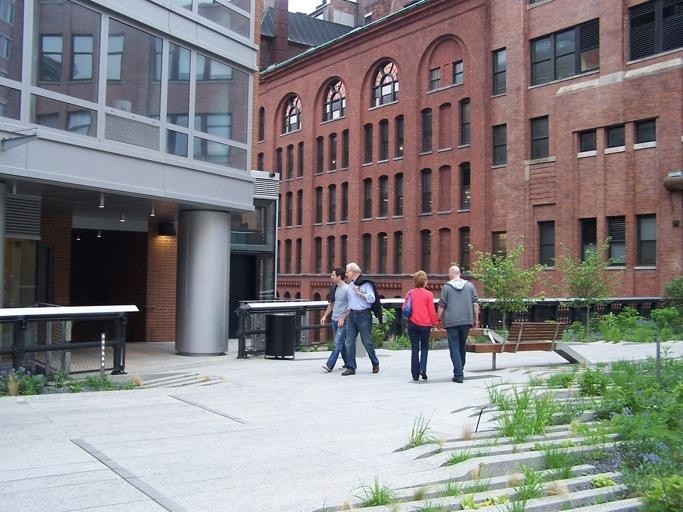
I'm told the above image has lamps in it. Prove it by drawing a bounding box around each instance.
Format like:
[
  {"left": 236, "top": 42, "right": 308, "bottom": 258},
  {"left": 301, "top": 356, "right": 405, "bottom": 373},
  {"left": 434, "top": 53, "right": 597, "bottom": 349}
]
[{"left": 76, "top": 191, "right": 157, "bottom": 241}]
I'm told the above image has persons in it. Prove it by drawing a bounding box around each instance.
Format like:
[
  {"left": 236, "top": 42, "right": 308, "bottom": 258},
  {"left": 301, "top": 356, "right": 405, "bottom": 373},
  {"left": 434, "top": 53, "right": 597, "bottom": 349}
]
[
  {"left": 339, "top": 262, "right": 382, "bottom": 376},
  {"left": 437, "top": 266, "right": 479, "bottom": 383},
  {"left": 400, "top": 270, "right": 437, "bottom": 382},
  {"left": 319, "top": 266, "right": 347, "bottom": 373}
]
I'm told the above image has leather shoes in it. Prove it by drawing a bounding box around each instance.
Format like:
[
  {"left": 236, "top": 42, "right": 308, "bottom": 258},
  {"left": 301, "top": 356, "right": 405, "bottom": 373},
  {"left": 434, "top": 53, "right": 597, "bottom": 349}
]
[{"left": 373, "top": 363, "right": 379, "bottom": 372}]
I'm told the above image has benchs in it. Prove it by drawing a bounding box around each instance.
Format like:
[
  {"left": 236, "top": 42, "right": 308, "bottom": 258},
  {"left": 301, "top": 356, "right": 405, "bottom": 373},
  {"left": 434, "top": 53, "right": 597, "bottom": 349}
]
[
  {"left": 463, "top": 322, "right": 566, "bottom": 373},
  {"left": 427, "top": 327, "right": 484, "bottom": 350}
]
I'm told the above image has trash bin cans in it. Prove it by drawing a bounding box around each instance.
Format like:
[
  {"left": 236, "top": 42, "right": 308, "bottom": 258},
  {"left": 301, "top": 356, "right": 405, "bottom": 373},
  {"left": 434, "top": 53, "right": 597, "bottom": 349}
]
[{"left": 264, "top": 313, "right": 296, "bottom": 359}]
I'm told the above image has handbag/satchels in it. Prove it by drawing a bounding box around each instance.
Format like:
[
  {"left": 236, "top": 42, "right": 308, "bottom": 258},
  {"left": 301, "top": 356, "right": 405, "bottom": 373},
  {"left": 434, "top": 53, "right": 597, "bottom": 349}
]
[{"left": 402, "top": 297, "right": 412, "bottom": 317}]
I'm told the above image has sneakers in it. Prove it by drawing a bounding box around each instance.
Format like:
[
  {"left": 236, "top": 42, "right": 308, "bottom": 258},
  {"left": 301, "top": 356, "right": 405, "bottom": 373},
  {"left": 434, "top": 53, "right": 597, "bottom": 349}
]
[
  {"left": 419, "top": 368, "right": 426, "bottom": 379},
  {"left": 321, "top": 363, "right": 355, "bottom": 375}
]
